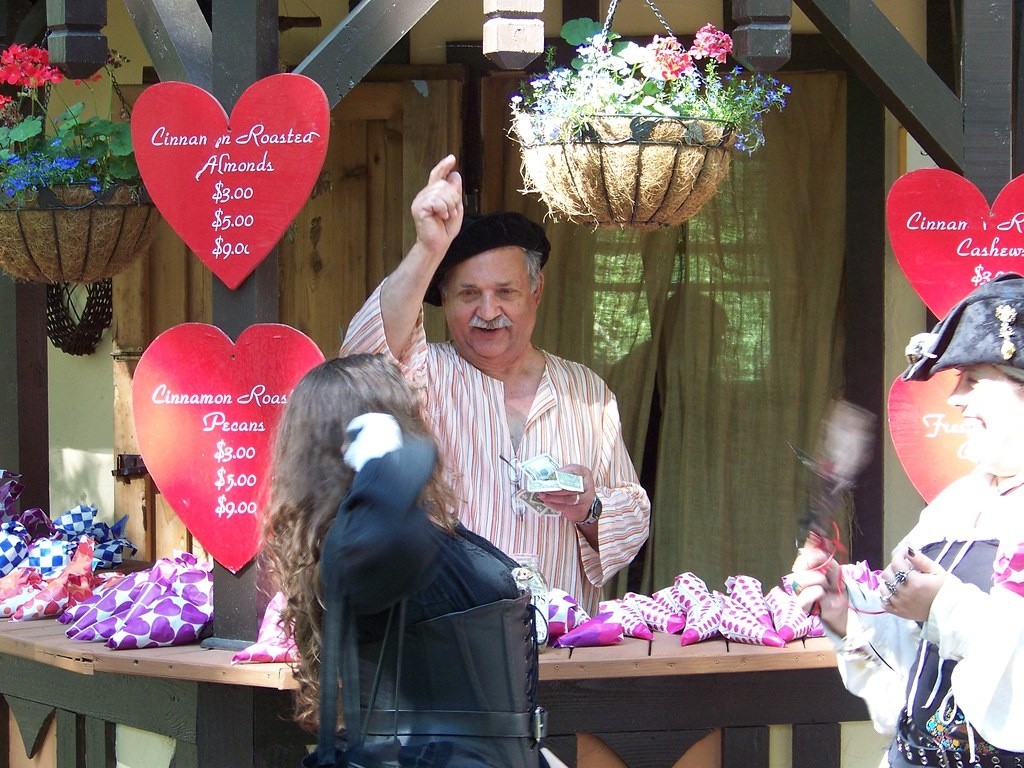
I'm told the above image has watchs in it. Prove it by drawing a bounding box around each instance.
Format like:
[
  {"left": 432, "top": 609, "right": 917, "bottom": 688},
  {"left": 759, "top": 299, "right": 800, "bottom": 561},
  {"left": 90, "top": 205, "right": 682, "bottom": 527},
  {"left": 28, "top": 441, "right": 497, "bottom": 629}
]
[{"left": 575, "top": 495, "right": 602, "bottom": 525}]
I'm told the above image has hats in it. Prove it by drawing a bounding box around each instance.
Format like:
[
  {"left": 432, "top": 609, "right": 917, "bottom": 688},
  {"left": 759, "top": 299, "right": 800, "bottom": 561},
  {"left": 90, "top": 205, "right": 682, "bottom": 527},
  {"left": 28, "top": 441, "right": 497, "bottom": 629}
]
[
  {"left": 421, "top": 211, "right": 551, "bottom": 307},
  {"left": 902, "top": 273, "right": 1024, "bottom": 382}
]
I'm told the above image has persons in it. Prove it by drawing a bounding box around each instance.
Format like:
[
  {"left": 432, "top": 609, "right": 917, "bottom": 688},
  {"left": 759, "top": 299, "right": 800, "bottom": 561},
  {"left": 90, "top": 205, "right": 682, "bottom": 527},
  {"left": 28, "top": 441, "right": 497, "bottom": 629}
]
[
  {"left": 340, "top": 155, "right": 652, "bottom": 767},
  {"left": 268, "top": 353, "right": 545, "bottom": 768},
  {"left": 792, "top": 273, "right": 1024, "bottom": 768}
]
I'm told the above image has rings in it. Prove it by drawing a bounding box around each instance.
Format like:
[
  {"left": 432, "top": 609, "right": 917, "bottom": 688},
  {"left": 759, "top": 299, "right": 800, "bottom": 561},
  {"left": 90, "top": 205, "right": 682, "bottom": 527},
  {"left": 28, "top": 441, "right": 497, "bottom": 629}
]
[
  {"left": 895, "top": 570, "right": 911, "bottom": 583},
  {"left": 885, "top": 581, "right": 897, "bottom": 593},
  {"left": 880, "top": 593, "right": 893, "bottom": 605},
  {"left": 572, "top": 495, "right": 579, "bottom": 506},
  {"left": 792, "top": 581, "right": 803, "bottom": 594}
]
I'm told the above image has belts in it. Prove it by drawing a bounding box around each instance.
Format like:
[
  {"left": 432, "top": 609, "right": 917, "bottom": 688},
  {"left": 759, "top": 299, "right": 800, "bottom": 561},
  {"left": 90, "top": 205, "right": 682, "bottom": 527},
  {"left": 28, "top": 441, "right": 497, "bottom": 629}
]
[{"left": 359, "top": 707, "right": 549, "bottom": 750}]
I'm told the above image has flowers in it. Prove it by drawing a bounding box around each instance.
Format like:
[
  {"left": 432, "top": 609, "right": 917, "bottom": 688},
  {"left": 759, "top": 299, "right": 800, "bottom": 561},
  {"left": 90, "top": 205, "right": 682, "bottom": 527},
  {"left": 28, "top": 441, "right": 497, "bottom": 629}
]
[
  {"left": 0, "top": 40, "right": 162, "bottom": 282},
  {"left": 505, "top": 16, "right": 793, "bottom": 229}
]
